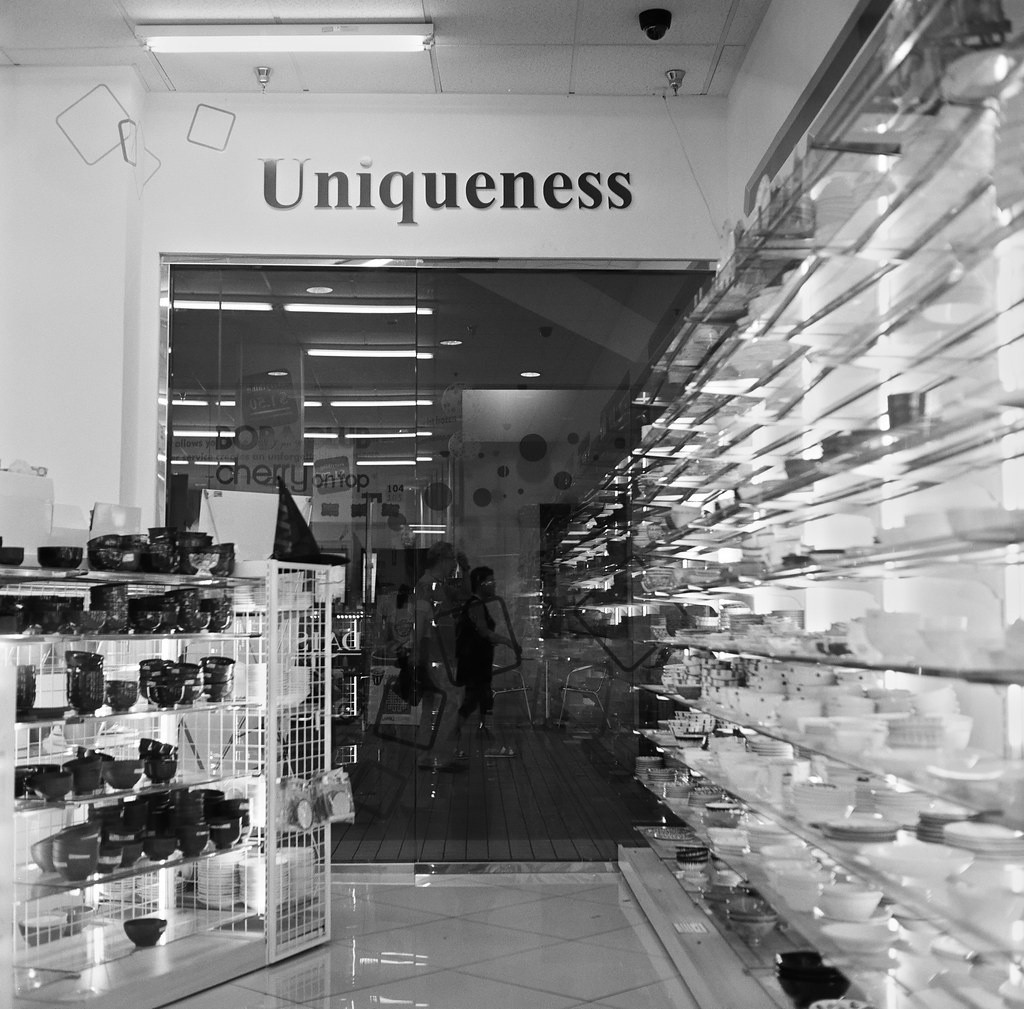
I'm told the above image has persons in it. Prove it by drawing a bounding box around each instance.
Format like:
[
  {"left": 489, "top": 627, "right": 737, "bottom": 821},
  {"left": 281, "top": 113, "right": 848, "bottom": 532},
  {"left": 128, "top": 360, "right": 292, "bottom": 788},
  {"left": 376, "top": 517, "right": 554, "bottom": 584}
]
[
  {"left": 453, "top": 567, "right": 523, "bottom": 761},
  {"left": 383, "top": 584, "right": 413, "bottom": 656},
  {"left": 408, "top": 541, "right": 473, "bottom": 772}
]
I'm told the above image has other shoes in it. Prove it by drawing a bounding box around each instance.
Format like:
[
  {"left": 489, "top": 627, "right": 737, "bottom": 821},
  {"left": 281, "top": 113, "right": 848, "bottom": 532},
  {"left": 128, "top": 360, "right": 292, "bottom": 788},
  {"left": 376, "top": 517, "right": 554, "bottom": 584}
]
[
  {"left": 434, "top": 755, "right": 470, "bottom": 777},
  {"left": 417, "top": 752, "right": 441, "bottom": 771}
]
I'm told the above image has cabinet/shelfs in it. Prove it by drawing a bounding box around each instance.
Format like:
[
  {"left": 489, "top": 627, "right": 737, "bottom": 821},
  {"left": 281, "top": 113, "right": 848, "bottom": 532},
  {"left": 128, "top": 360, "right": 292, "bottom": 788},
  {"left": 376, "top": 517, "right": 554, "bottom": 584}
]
[
  {"left": 538, "top": 440, "right": 640, "bottom": 633},
  {"left": 608, "top": 0, "right": 1023, "bottom": 1009},
  {"left": 1, "top": 556, "right": 335, "bottom": 1009}
]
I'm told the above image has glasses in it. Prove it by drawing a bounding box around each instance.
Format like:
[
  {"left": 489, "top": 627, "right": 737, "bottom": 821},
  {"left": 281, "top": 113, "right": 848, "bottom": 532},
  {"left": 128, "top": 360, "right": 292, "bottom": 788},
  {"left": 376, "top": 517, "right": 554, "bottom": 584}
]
[
  {"left": 438, "top": 553, "right": 458, "bottom": 561},
  {"left": 479, "top": 578, "right": 499, "bottom": 588}
]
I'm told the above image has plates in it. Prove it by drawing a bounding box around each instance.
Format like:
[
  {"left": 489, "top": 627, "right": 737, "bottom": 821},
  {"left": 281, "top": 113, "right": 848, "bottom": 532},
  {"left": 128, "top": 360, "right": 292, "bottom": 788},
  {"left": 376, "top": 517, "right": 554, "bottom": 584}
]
[{"left": 196, "top": 560, "right": 319, "bottom": 913}]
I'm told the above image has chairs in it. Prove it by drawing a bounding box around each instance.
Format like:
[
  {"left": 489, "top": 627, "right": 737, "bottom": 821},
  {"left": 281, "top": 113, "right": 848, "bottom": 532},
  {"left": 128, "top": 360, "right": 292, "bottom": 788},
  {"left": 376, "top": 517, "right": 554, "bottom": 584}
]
[
  {"left": 492, "top": 664, "right": 535, "bottom": 727},
  {"left": 558, "top": 658, "right": 613, "bottom": 730}
]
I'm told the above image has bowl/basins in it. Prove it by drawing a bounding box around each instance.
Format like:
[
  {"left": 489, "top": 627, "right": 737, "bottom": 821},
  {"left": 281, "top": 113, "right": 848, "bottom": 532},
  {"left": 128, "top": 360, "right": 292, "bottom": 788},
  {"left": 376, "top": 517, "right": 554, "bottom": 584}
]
[
  {"left": 650, "top": 2, "right": 1023, "bottom": 1009},
  {"left": 0, "top": 526, "right": 252, "bottom": 949}
]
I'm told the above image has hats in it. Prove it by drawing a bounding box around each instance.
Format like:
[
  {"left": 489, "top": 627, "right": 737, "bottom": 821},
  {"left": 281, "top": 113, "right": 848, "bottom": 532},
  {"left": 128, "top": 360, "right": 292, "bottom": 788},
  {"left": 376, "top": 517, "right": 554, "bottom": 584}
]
[{"left": 420, "top": 540, "right": 455, "bottom": 567}]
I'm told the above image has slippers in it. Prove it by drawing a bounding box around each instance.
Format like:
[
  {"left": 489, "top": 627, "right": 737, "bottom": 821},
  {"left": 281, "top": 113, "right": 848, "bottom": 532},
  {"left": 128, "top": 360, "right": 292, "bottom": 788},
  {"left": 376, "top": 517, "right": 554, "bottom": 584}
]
[
  {"left": 483, "top": 743, "right": 522, "bottom": 760},
  {"left": 454, "top": 745, "right": 482, "bottom": 760}
]
